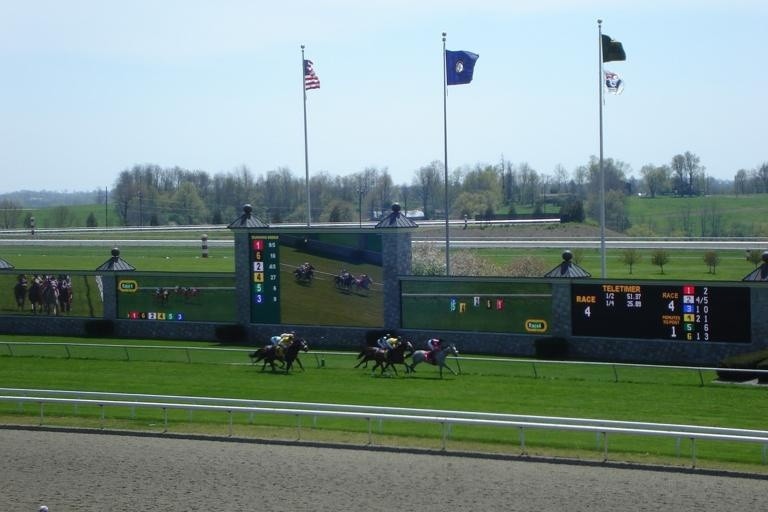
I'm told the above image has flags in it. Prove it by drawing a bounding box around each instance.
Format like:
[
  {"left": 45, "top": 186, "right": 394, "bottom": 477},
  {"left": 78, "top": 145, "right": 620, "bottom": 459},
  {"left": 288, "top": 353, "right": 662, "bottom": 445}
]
[
  {"left": 446, "top": 50, "right": 479, "bottom": 85},
  {"left": 602, "top": 34, "right": 626, "bottom": 63},
  {"left": 304, "top": 59, "right": 320, "bottom": 90},
  {"left": 604, "top": 72, "right": 625, "bottom": 96}
]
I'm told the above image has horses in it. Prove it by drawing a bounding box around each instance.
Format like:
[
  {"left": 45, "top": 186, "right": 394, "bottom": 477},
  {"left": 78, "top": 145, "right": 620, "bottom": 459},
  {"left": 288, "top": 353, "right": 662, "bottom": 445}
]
[
  {"left": 407, "top": 343, "right": 458, "bottom": 379},
  {"left": 249, "top": 337, "right": 309, "bottom": 375},
  {"left": 334, "top": 274, "right": 374, "bottom": 296},
  {"left": 354, "top": 339, "right": 415, "bottom": 375},
  {"left": 13, "top": 278, "right": 73, "bottom": 317},
  {"left": 292, "top": 264, "right": 316, "bottom": 285}
]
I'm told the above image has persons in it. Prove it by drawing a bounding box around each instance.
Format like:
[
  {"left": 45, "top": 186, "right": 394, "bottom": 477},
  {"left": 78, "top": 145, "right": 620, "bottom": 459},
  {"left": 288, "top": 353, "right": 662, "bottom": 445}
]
[
  {"left": 377, "top": 333, "right": 402, "bottom": 361},
  {"left": 334, "top": 268, "right": 370, "bottom": 290},
  {"left": 296, "top": 260, "right": 311, "bottom": 280},
  {"left": 12, "top": 274, "right": 73, "bottom": 301},
  {"left": 263, "top": 329, "right": 298, "bottom": 363},
  {"left": 427, "top": 338, "right": 444, "bottom": 365}
]
